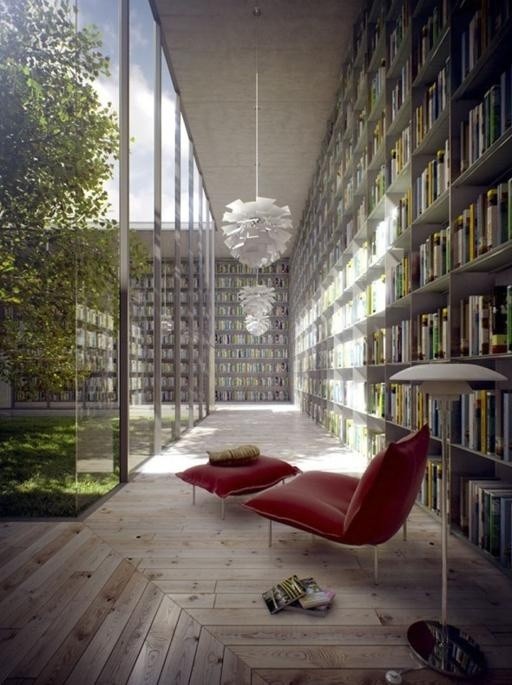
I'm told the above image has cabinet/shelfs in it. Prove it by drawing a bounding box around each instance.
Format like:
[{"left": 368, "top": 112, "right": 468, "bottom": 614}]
[
  {"left": 41, "top": 255, "right": 206, "bottom": 408},
  {"left": 214, "top": 257, "right": 289, "bottom": 402},
  {"left": 288, "top": 1, "right": 512, "bottom": 575}
]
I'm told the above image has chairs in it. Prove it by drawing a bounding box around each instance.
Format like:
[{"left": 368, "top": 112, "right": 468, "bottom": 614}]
[{"left": 241, "top": 423, "right": 432, "bottom": 585}]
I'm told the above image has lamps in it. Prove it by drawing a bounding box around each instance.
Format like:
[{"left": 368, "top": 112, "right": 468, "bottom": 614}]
[
  {"left": 239, "top": 106, "right": 277, "bottom": 318},
  {"left": 388, "top": 364, "right": 509, "bottom": 678},
  {"left": 222, "top": 7, "right": 293, "bottom": 268},
  {"left": 244, "top": 266, "right": 272, "bottom": 335}
]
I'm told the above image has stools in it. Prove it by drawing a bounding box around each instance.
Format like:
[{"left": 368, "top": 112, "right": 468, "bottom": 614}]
[{"left": 175, "top": 445, "right": 302, "bottom": 520}]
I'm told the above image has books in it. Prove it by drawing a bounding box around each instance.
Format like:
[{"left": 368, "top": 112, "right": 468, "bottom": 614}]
[
  {"left": 369, "top": 164, "right": 389, "bottom": 211},
  {"left": 391, "top": 57, "right": 412, "bottom": 123},
  {"left": 311, "top": 106, "right": 352, "bottom": 184},
  {"left": 261, "top": 573, "right": 334, "bottom": 616},
  {"left": 352, "top": 422, "right": 368, "bottom": 455},
  {"left": 367, "top": 428, "right": 385, "bottom": 459},
  {"left": 354, "top": 106, "right": 368, "bottom": 142},
  {"left": 413, "top": 383, "right": 451, "bottom": 440},
  {"left": 416, "top": 305, "right": 447, "bottom": 361},
  {"left": 420, "top": 454, "right": 442, "bottom": 520},
  {"left": 215, "top": 389, "right": 288, "bottom": 401},
  {"left": 287, "top": 241, "right": 352, "bottom": 433},
  {"left": 369, "top": 328, "right": 385, "bottom": 365},
  {"left": 354, "top": 289, "right": 370, "bottom": 322},
  {"left": 388, "top": 190, "right": 412, "bottom": 244},
  {"left": 215, "top": 318, "right": 286, "bottom": 330},
  {"left": 216, "top": 275, "right": 287, "bottom": 287},
  {"left": 369, "top": 57, "right": 388, "bottom": 110},
  {"left": 460, "top": 389, "right": 510, "bottom": 462},
  {"left": 370, "top": 273, "right": 386, "bottom": 313},
  {"left": 388, "top": 382, "right": 413, "bottom": 429},
  {"left": 215, "top": 305, "right": 286, "bottom": 316},
  {"left": 6, "top": 259, "right": 207, "bottom": 407},
  {"left": 367, "top": 382, "right": 385, "bottom": 418},
  {"left": 390, "top": 120, "right": 413, "bottom": 184},
  {"left": 414, "top": 58, "right": 450, "bottom": 148},
  {"left": 452, "top": 1, "right": 511, "bottom": 86},
  {"left": 414, "top": 140, "right": 448, "bottom": 217},
  {"left": 352, "top": 333, "right": 366, "bottom": 367},
  {"left": 416, "top": 1, "right": 449, "bottom": 64},
  {"left": 390, "top": 320, "right": 410, "bottom": 364},
  {"left": 388, "top": 1, "right": 412, "bottom": 66},
  {"left": 337, "top": 15, "right": 367, "bottom": 104},
  {"left": 296, "top": 184, "right": 355, "bottom": 240},
  {"left": 216, "top": 361, "right": 286, "bottom": 373},
  {"left": 215, "top": 332, "right": 287, "bottom": 345},
  {"left": 366, "top": 15, "right": 384, "bottom": 56},
  {"left": 371, "top": 219, "right": 388, "bottom": 265},
  {"left": 368, "top": 114, "right": 387, "bottom": 158},
  {"left": 354, "top": 146, "right": 371, "bottom": 189},
  {"left": 457, "top": 476, "right": 511, "bottom": 568},
  {"left": 389, "top": 256, "right": 409, "bottom": 303},
  {"left": 419, "top": 226, "right": 452, "bottom": 287},
  {"left": 459, "top": 72, "right": 510, "bottom": 174},
  {"left": 351, "top": 382, "right": 366, "bottom": 412},
  {"left": 215, "top": 375, "right": 287, "bottom": 387},
  {"left": 213, "top": 287, "right": 286, "bottom": 301},
  {"left": 356, "top": 193, "right": 370, "bottom": 231},
  {"left": 216, "top": 263, "right": 286, "bottom": 272},
  {"left": 356, "top": 241, "right": 367, "bottom": 277},
  {"left": 216, "top": 347, "right": 288, "bottom": 358},
  {"left": 458, "top": 284, "right": 511, "bottom": 357},
  {"left": 456, "top": 183, "right": 507, "bottom": 266},
  {"left": 343, "top": 418, "right": 353, "bottom": 447}
]
